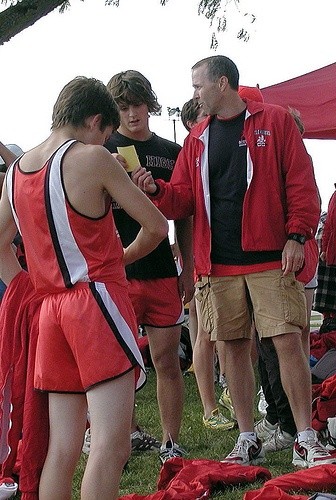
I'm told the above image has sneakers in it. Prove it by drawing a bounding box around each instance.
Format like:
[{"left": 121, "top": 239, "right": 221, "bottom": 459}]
[
  {"left": 82, "top": 427, "right": 162, "bottom": 456},
  {"left": 201, "top": 408, "right": 236, "bottom": 431},
  {"left": 218, "top": 386, "right": 237, "bottom": 420},
  {"left": 221, "top": 435, "right": 268, "bottom": 466},
  {"left": 291, "top": 431, "right": 336, "bottom": 468},
  {"left": 252, "top": 385, "right": 297, "bottom": 454},
  {"left": 159, "top": 442, "right": 185, "bottom": 465}
]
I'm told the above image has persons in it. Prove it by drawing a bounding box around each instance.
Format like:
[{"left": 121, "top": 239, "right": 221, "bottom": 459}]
[
  {"left": 131, "top": 55, "right": 336, "bottom": 469},
  {"left": 0, "top": 74, "right": 170, "bottom": 500},
  {"left": 180, "top": 85, "right": 336, "bottom": 455},
  {"left": 0, "top": 140, "right": 163, "bottom": 500},
  {"left": 106, "top": 70, "right": 195, "bottom": 466}
]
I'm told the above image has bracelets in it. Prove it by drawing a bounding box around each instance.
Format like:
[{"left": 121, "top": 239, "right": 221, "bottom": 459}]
[{"left": 286, "top": 233, "right": 306, "bottom": 246}]
